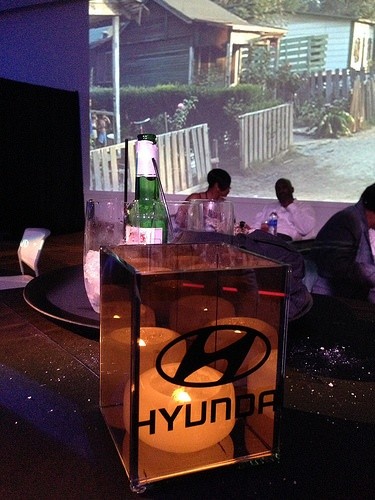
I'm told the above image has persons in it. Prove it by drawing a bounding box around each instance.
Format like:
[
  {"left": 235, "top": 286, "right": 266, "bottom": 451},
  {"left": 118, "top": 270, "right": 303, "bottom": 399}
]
[
  {"left": 252, "top": 177, "right": 314, "bottom": 245},
  {"left": 173, "top": 168, "right": 231, "bottom": 230},
  {"left": 311, "top": 183, "right": 375, "bottom": 303}
]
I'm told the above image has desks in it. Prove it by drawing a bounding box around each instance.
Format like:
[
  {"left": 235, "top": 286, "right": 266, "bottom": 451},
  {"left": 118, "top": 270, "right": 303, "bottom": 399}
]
[{"left": 0, "top": 287, "right": 375, "bottom": 500}]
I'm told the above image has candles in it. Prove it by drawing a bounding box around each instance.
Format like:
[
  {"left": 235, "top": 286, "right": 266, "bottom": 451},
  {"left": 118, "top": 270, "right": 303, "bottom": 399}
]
[{"left": 121, "top": 355, "right": 237, "bottom": 455}]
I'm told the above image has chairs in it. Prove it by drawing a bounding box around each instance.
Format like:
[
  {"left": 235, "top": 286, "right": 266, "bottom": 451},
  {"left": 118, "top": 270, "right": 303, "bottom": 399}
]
[{"left": 0, "top": 228, "right": 51, "bottom": 291}]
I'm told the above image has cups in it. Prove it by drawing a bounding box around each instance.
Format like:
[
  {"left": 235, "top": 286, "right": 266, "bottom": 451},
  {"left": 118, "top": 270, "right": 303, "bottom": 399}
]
[{"left": 83, "top": 193, "right": 235, "bottom": 313}]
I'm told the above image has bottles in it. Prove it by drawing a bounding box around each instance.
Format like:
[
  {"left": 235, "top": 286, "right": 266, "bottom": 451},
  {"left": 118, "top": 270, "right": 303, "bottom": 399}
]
[
  {"left": 123, "top": 133, "right": 168, "bottom": 243},
  {"left": 268, "top": 209, "right": 278, "bottom": 236}
]
